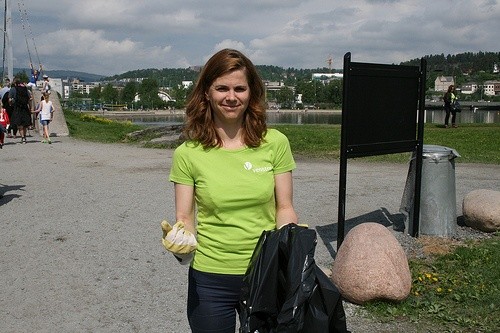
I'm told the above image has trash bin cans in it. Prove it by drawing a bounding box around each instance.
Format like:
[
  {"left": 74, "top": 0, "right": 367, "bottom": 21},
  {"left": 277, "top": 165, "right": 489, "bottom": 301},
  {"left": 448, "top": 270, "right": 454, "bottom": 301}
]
[{"left": 409, "top": 145, "right": 457, "bottom": 241}]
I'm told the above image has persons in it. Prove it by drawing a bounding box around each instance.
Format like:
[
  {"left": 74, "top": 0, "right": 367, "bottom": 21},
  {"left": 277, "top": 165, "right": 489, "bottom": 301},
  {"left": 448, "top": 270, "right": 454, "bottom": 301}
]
[
  {"left": 30, "top": 92, "right": 55, "bottom": 144},
  {"left": 0, "top": 75, "right": 51, "bottom": 149},
  {"left": 29, "top": 63, "right": 42, "bottom": 83},
  {"left": 160, "top": 48, "right": 298, "bottom": 333},
  {"left": 443, "top": 85, "right": 458, "bottom": 128}
]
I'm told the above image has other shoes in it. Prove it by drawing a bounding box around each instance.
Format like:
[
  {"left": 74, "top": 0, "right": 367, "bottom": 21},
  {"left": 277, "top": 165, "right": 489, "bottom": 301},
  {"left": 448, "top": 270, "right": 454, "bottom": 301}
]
[
  {"left": 452, "top": 124, "right": 458, "bottom": 128},
  {"left": 14, "top": 135, "right": 16, "bottom": 139},
  {"left": 47, "top": 139, "right": 52, "bottom": 144},
  {"left": 22, "top": 137, "right": 26, "bottom": 142},
  {"left": 42, "top": 138, "right": 46, "bottom": 143},
  {"left": 6, "top": 133, "right": 12, "bottom": 138},
  {"left": 444, "top": 125, "right": 449, "bottom": 128}
]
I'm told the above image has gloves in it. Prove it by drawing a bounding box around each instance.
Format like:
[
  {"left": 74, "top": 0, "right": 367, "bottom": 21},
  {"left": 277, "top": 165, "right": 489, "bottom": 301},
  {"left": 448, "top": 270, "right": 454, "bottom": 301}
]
[{"left": 161, "top": 220, "right": 198, "bottom": 254}]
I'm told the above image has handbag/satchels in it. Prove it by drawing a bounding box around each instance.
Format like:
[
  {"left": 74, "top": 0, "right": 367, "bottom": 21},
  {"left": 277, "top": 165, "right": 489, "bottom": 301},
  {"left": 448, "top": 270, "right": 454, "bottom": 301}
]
[{"left": 452, "top": 98, "right": 461, "bottom": 112}]
